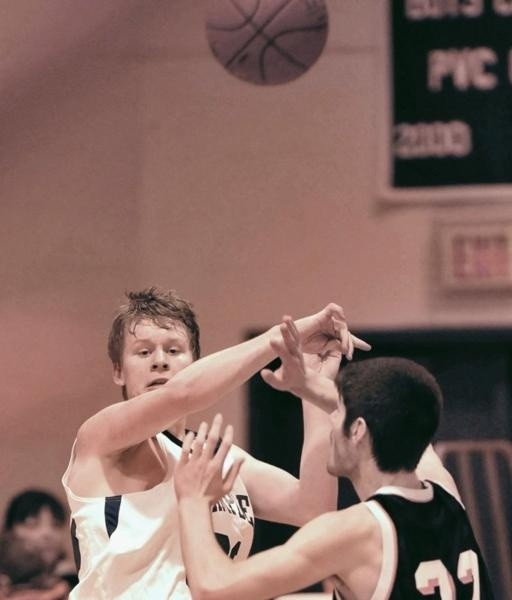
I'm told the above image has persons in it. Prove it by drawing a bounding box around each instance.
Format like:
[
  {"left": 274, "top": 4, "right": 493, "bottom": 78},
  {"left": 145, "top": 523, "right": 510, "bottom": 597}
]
[
  {"left": 172, "top": 314, "right": 496, "bottom": 599},
  {"left": 59, "top": 284, "right": 372, "bottom": 599},
  {"left": 0, "top": 489, "right": 71, "bottom": 600}
]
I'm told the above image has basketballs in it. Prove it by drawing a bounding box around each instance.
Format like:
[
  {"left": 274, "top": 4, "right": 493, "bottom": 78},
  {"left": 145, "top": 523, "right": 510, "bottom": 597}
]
[{"left": 205, "top": 0, "right": 328, "bottom": 87}]
